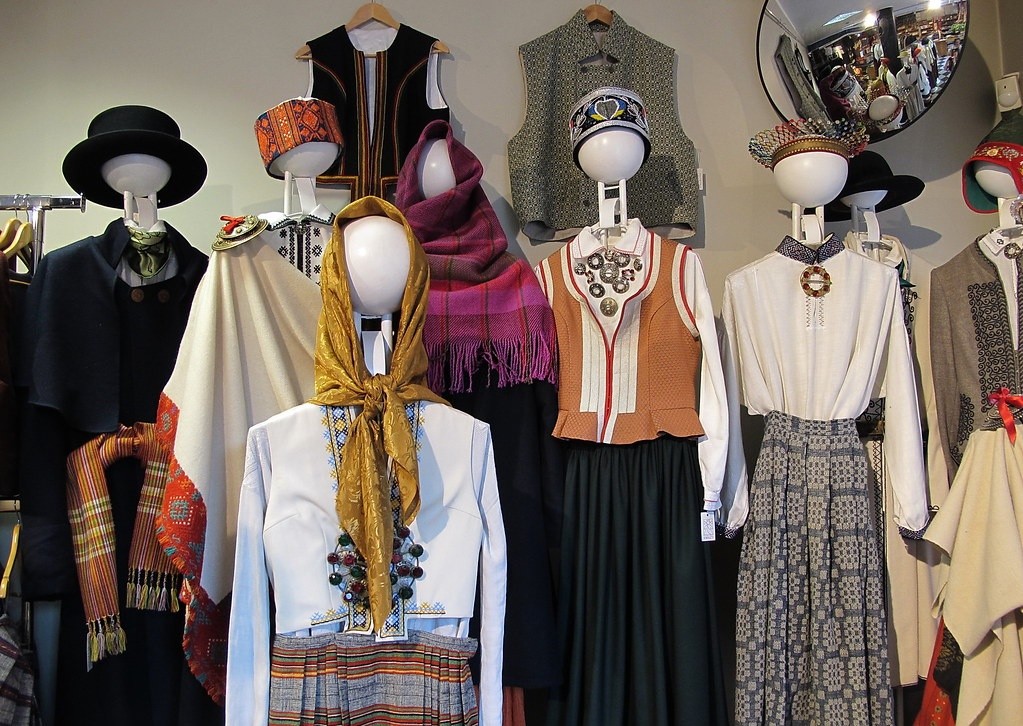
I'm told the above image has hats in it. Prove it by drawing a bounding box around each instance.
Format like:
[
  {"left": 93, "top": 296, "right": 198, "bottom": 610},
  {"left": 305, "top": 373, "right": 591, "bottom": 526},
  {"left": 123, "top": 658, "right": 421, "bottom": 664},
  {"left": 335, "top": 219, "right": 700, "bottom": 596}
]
[
  {"left": 914, "top": 48, "right": 922, "bottom": 57},
  {"left": 897, "top": 51, "right": 909, "bottom": 58},
  {"left": 880, "top": 58, "right": 890, "bottom": 66},
  {"left": 61, "top": 105, "right": 207, "bottom": 209},
  {"left": 568, "top": 86, "right": 651, "bottom": 178},
  {"left": 962, "top": 112, "right": 1023, "bottom": 215},
  {"left": 254, "top": 97, "right": 345, "bottom": 180},
  {"left": 803, "top": 150, "right": 925, "bottom": 222}
]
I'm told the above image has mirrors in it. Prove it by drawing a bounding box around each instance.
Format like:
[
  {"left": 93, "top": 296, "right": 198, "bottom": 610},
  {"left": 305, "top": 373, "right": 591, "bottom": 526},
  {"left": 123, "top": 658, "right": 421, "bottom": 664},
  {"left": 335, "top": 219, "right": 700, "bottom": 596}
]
[{"left": 756, "top": 0, "right": 970, "bottom": 145}]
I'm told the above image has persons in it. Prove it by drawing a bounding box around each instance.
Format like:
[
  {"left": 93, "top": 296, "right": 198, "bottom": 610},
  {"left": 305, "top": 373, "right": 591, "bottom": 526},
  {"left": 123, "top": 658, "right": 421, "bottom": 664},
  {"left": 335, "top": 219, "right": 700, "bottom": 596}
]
[
  {"left": 392, "top": 118, "right": 559, "bottom": 702},
  {"left": 222, "top": 195, "right": 508, "bottom": 726},
  {"left": 715, "top": 119, "right": 953, "bottom": 725},
  {"left": 154, "top": 96, "right": 342, "bottom": 726},
  {"left": 919, "top": 112, "right": 1023, "bottom": 726},
  {"left": 529, "top": 87, "right": 730, "bottom": 726},
  {"left": 878, "top": 39, "right": 939, "bottom": 121},
  {"left": 7, "top": 100, "right": 211, "bottom": 726}
]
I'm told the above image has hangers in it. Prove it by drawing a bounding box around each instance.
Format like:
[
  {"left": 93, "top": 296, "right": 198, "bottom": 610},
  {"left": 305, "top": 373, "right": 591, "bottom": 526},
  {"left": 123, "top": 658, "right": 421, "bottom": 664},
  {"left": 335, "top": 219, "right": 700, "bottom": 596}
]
[
  {"left": 0, "top": 494, "right": 20, "bottom": 599},
  {"left": 0, "top": 193, "right": 45, "bottom": 286}
]
